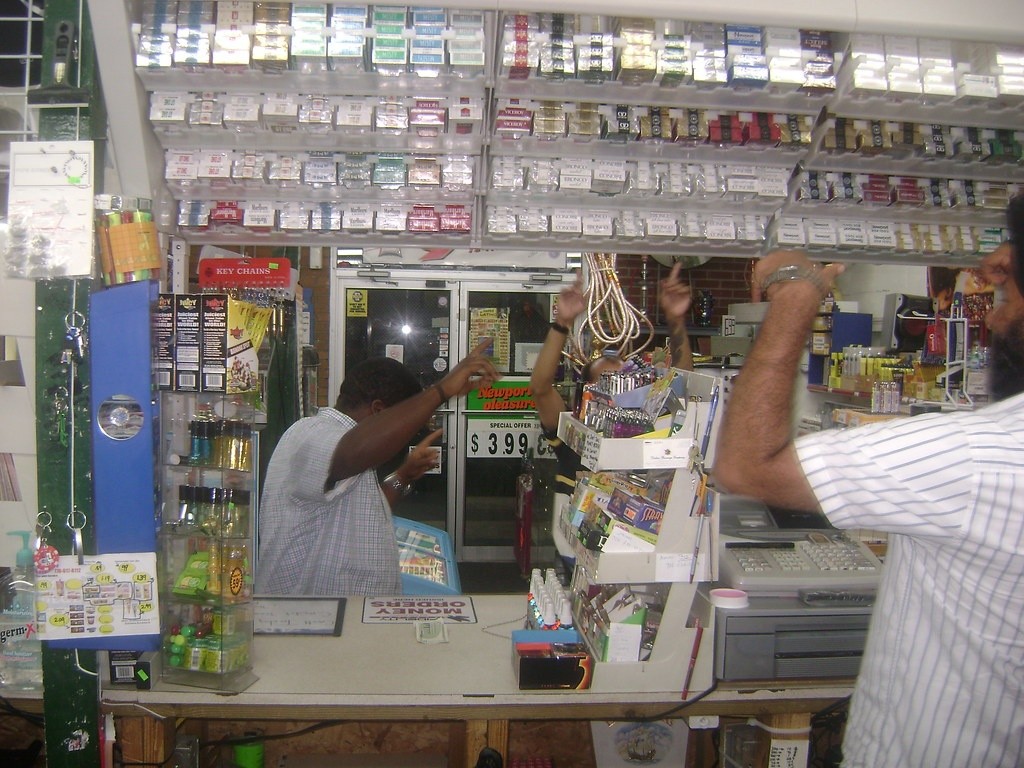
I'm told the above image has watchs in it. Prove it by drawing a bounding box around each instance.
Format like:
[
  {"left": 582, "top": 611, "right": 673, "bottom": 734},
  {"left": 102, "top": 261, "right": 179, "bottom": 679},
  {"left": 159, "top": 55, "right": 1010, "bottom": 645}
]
[
  {"left": 760, "top": 264, "right": 827, "bottom": 302},
  {"left": 384, "top": 474, "right": 411, "bottom": 496}
]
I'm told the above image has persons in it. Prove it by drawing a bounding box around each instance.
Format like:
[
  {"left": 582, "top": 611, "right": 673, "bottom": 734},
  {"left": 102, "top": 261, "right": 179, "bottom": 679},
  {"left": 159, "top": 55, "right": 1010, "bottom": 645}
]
[
  {"left": 530, "top": 261, "right": 694, "bottom": 582},
  {"left": 254, "top": 338, "right": 502, "bottom": 596},
  {"left": 710, "top": 194, "right": 1024, "bottom": 768}
]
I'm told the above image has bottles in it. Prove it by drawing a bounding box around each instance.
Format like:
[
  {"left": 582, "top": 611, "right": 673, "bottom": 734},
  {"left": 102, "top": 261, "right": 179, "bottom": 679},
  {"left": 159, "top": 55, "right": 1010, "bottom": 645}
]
[
  {"left": 179, "top": 485, "right": 250, "bottom": 537},
  {"left": 206, "top": 538, "right": 248, "bottom": 598},
  {"left": 190, "top": 419, "right": 251, "bottom": 472}
]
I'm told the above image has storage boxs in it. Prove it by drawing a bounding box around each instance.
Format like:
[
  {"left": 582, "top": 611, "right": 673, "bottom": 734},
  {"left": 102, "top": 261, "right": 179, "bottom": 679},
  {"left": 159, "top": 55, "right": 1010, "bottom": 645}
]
[
  {"left": 133, "top": 0, "right": 1024, "bottom": 255},
  {"left": 560, "top": 367, "right": 687, "bottom": 662}
]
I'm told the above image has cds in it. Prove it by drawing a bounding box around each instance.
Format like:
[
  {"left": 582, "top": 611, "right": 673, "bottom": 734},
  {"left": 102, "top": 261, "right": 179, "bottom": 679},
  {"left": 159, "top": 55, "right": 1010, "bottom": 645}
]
[{"left": 98, "top": 394, "right": 144, "bottom": 441}]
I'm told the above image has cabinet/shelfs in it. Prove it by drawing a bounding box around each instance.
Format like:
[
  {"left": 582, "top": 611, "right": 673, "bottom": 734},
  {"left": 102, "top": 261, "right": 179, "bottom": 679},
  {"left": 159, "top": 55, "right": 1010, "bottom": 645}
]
[
  {"left": 149, "top": 0, "right": 498, "bottom": 254},
  {"left": 805, "top": 312, "right": 873, "bottom": 387},
  {"left": 557, "top": 367, "right": 726, "bottom": 690},
  {"left": 158, "top": 389, "right": 259, "bottom": 697}
]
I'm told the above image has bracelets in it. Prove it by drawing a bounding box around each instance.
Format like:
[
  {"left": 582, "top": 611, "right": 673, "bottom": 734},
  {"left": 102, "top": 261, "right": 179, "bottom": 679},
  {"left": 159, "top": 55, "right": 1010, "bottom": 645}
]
[
  {"left": 551, "top": 322, "right": 569, "bottom": 334},
  {"left": 434, "top": 384, "right": 445, "bottom": 404}
]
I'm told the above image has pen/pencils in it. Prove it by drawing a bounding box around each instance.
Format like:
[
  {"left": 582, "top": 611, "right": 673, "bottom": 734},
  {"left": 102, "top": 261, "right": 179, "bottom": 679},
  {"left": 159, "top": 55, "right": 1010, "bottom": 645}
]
[
  {"left": 681, "top": 627, "right": 704, "bottom": 700},
  {"left": 706, "top": 492, "right": 714, "bottom": 584},
  {"left": 701, "top": 385, "right": 719, "bottom": 460},
  {"left": 690, "top": 506, "right": 705, "bottom": 584},
  {"left": 725, "top": 542, "right": 795, "bottom": 550}
]
